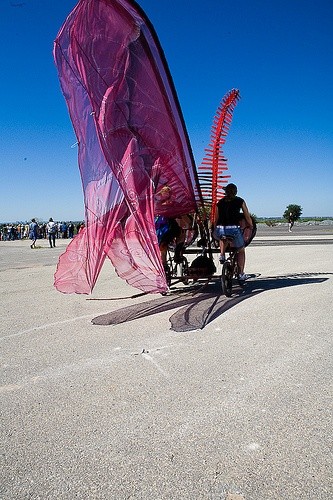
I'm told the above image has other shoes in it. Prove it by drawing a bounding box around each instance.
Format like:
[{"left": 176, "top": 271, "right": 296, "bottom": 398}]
[{"left": 174, "top": 255, "right": 185, "bottom": 262}]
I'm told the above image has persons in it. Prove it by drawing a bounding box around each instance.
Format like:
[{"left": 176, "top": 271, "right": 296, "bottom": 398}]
[
  {"left": 29, "top": 219, "right": 39, "bottom": 248},
  {"left": 45, "top": 218, "right": 57, "bottom": 248},
  {"left": 289, "top": 212, "right": 294, "bottom": 233},
  {"left": 217, "top": 182, "right": 254, "bottom": 281},
  {"left": 0, "top": 220, "right": 86, "bottom": 241},
  {"left": 152, "top": 187, "right": 187, "bottom": 273}
]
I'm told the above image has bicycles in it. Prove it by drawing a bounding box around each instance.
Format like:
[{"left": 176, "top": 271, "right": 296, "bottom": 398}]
[{"left": 157, "top": 223, "right": 257, "bottom": 298}]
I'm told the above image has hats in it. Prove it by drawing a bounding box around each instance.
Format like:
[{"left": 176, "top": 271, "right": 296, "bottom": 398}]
[{"left": 222, "top": 184, "right": 237, "bottom": 194}]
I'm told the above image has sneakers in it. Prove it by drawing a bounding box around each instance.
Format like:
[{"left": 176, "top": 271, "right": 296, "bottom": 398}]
[
  {"left": 239, "top": 273, "right": 247, "bottom": 280},
  {"left": 219, "top": 256, "right": 225, "bottom": 264}
]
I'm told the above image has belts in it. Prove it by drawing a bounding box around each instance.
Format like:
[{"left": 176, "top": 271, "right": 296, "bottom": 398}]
[{"left": 216, "top": 226, "right": 240, "bottom": 229}]
